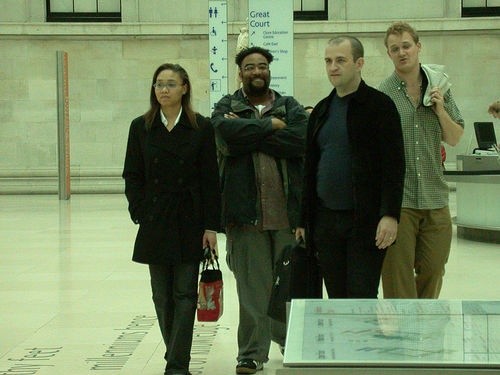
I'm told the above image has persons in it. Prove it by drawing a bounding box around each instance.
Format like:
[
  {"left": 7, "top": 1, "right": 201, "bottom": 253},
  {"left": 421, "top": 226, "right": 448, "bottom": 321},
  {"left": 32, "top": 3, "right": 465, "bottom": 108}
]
[
  {"left": 123, "top": 64, "right": 217, "bottom": 375},
  {"left": 296, "top": 35, "right": 405, "bottom": 299},
  {"left": 377, "top": 22, "right": 464, "bottom": 299},
  {"left": 213, "top": 47, "right": 309, "bottom": 374}
]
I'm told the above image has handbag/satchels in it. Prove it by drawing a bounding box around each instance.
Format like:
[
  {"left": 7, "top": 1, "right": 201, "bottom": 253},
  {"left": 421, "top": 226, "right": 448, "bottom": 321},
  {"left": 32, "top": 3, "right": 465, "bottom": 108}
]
[{"left": 197, "top": 246, "right": 224, "bottom": 322}]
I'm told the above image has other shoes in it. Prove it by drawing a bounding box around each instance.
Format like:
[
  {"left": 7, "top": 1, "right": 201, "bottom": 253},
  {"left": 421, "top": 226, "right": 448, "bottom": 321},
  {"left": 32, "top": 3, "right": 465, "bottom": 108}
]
[{"left": 235, "top": 359, "right": 265, "bottom": 374}]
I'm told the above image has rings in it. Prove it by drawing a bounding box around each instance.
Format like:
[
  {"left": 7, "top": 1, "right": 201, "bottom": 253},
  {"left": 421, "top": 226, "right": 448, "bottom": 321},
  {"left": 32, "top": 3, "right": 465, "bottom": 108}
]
[{"left": 390, "top": 237, "right": 393, "bottom": 240}]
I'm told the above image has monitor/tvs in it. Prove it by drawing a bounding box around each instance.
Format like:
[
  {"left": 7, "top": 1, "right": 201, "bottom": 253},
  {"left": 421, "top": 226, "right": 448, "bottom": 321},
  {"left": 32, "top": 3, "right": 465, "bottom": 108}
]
[{"left": 474, "top": 122, "right": 497, "bottom": 150}]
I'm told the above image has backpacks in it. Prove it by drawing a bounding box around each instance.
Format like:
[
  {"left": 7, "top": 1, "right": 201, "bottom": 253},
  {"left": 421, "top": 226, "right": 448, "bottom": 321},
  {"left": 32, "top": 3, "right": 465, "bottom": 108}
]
[{"left": 268, "top": 239, "right": 315, "bottom": 321}]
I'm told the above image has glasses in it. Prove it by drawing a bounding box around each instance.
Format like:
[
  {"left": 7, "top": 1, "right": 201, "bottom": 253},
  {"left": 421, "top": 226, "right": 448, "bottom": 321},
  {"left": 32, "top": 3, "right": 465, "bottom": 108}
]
[
  {"left": 240, "top": 64, "right": 269, "bottom": 71},
  {"left": 153, "top": 82, "right": 184, "bottom": 89}
]
